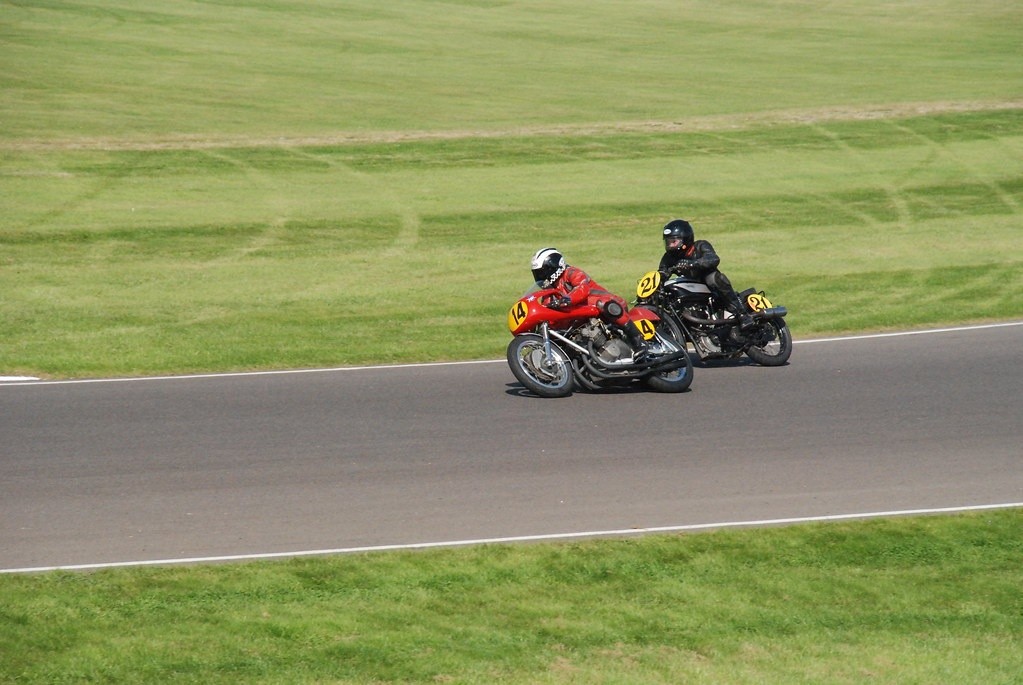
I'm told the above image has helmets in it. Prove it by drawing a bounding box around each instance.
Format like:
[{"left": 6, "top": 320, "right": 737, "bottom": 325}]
[
  {"left": 531, "top": 248, "right": 566, "bottom": 289},
  {"left": 663, "top": 220, "right": 694, "bottom": 259}
]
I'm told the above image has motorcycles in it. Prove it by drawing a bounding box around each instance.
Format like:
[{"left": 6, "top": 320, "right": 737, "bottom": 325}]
[
  {"left": 506, "top": 280, "right": 695, "bottom": 397},
  {"left": 631, "top": 272, "right": 795, "bottom": 366}
]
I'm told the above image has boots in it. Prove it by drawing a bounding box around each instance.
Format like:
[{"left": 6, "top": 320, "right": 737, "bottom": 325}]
[
  {"left": 728, "top": 296, "right": 754, "bottom": 332},
  {"left": 624, "top": 319, "right": 649, "bottom": 357}
]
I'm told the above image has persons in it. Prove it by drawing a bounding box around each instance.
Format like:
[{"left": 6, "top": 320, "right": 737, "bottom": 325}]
[
  {"left": 660, "top": 219, "right": 755, "bottom": 358},
  {"left": 531, "top": 248, "right": 648, "bottom": 364}
]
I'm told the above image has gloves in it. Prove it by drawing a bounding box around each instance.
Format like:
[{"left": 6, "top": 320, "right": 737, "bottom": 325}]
[
  {"left": 674, "top": 259, "right": 700, "bottom": 276},
  {"left": 546, "top": 296, "right": 572, "bottom": 310}
]
